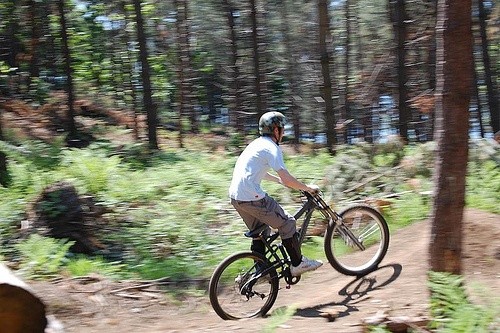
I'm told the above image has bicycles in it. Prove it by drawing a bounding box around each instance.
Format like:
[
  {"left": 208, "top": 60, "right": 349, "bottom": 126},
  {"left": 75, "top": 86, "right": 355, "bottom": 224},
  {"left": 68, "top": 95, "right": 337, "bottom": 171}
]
[{"left": 207, "top": 184, "right": 389, "bottom": 322}]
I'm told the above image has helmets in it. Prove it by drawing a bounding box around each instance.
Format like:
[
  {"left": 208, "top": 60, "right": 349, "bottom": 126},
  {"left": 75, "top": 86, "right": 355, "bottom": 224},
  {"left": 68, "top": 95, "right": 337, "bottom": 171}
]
[{"left": 258, "top": 111, "right": 287, "bottom": 134}]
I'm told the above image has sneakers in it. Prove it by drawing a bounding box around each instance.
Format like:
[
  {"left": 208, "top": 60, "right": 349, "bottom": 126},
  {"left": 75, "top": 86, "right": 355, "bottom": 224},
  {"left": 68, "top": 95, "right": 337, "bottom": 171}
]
[
  {"left": 255, "top": 272, "right": 281, "bottom": 283},
  {"left": 290, "top": 255, "right": 323, "bottom": 276}
]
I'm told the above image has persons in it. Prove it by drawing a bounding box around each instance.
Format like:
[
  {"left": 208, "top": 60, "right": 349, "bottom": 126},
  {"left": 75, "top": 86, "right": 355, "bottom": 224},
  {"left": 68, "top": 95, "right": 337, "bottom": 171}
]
[{"left": 228, "top": 110, "right": 324, "bottom": 283}]
